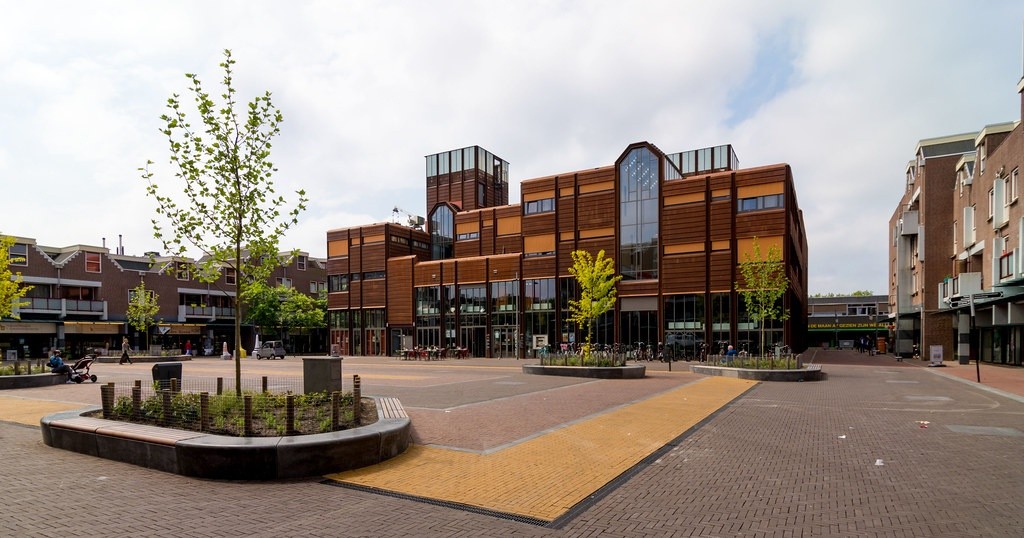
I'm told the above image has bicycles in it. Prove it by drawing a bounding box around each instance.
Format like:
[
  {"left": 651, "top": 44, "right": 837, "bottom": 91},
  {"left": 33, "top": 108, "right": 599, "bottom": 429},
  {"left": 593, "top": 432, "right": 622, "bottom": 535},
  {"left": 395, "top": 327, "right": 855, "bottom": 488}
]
[
  {"left": 700, "top": 343, "right": 709, "bottom": 361},
  {"left": 633, "top": 341, "right": 645, "bottom": 362},
  {"left": 658, "top": 343, "right": 665, "bottom": 362},
  {"left": 646, "top": 344, "right": 653, "bottom": 363}
]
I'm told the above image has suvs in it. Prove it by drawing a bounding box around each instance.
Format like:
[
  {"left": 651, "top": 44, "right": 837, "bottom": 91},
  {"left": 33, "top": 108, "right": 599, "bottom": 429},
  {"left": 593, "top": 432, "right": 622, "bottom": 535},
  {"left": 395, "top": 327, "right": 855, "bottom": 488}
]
[{"left": 668, "top": 335, "right": 702, "bottom": 348}]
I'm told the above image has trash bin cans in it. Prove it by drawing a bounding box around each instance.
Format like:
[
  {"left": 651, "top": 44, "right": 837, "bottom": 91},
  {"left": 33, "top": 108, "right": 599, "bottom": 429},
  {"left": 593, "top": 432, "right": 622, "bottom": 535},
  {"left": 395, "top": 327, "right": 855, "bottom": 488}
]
[
  {"left": 839, "top": 340, "right": 854, "bottom": 350},
  {"left": 152, "top": 362, "right": 182, "bottom": 394}
]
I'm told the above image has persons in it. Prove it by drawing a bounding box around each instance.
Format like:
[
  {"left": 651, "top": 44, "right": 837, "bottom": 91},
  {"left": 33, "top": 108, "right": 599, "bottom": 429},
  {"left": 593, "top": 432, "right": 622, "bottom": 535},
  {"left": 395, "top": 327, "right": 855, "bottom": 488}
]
[
  {"left": 119, "top": 337, "right": 135, "bottom": 365},
  {"left": 49, "top": 350, "right": 79, "bottom": 384},
  {"left": 185, "top": 340, "right": 193, "bottom": 355},
  {"left": 856, "top": 334, "right": 875, "bottom": 357},
  {"left": 719, "top": 344, "right": 738, "bottom": 364}
]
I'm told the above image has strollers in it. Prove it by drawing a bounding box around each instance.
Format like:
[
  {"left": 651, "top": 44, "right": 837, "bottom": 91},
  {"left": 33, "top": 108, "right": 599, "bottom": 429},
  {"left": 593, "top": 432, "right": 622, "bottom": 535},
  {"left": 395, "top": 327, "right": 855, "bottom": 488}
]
[{"left": 68, "top": 353, "right": 98, "bottom": 384}]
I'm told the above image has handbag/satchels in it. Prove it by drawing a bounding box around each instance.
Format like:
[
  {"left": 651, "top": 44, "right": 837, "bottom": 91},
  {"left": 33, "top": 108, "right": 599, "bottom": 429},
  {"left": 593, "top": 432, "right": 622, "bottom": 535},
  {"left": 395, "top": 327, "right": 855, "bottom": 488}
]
[
  {"left": 46, "top": 362, "right": 59, "bottom": 368},
  {"left": 120, "top": 353, "right": 127, "bottom": 363}
]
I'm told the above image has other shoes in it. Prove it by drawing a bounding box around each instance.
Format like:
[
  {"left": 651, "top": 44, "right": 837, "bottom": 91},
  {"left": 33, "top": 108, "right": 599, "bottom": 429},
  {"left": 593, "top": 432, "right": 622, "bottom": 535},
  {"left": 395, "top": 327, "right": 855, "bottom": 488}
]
[{"left": 66, "top": 379, "right": 77, "bottom": 384}]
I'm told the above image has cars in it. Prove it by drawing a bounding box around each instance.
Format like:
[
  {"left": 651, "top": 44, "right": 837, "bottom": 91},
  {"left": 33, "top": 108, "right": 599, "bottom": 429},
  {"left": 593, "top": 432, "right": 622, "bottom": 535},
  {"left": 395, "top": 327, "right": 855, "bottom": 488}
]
[
  {"left": 256, "top": 341, "right": 286, "bottom": 360},
  {"left": 716, "top": 340, "right": 780, "bottom": 355}
]
